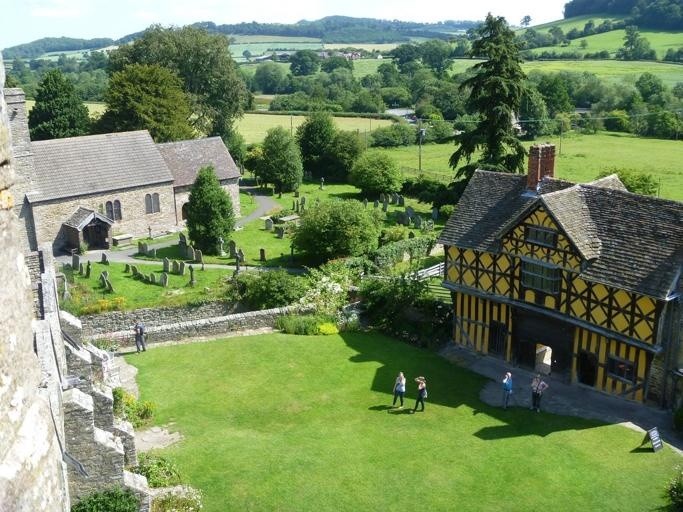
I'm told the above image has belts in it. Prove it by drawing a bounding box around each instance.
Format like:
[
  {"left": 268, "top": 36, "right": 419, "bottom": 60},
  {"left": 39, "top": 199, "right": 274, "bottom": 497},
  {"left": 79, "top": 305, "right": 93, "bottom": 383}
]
[{"left": 504, "top": 389, "right": 511, "bottom": 391}]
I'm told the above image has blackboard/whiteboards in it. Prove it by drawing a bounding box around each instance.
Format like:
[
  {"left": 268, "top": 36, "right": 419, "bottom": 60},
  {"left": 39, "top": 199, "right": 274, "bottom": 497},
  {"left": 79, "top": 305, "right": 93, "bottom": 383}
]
[{"left": 649, "top": 427, "right": 664, "bottom": 452}]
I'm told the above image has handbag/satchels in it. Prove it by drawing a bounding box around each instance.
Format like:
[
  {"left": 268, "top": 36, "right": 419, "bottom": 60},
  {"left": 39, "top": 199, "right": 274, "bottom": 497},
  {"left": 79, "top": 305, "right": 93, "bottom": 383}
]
[{"left": 423, "top": 389, "right": 428, "bottom": 398}]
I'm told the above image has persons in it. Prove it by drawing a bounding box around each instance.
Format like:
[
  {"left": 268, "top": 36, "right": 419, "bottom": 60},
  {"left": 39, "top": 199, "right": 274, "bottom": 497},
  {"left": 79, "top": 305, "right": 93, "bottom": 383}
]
[
  {"left": 409, "top": 375, "right": 427, "bottom": 414},
  {"left": 529, "top": 373, "right": 548, "bottom": 411},
  {"left": 391, "top": 370, "right": 406, "bottom": 408},
  {"left": 132, "top": 319, "right": 146, "bottom": 353},
  {"left": 501, "top": 371, "right": 511, "bottom": 409}
]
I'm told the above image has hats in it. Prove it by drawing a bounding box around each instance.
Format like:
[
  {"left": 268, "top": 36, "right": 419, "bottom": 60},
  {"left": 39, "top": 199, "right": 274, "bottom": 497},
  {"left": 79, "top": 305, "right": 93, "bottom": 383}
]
[{"left": 534, "top": 374, "right": 542, "bottom": 378}]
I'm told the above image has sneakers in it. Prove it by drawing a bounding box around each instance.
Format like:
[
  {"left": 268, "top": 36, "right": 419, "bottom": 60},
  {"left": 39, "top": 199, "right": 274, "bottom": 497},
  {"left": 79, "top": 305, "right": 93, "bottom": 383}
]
[{"left": 529, "top": 406, "right": 541, "bottom": 413}]
